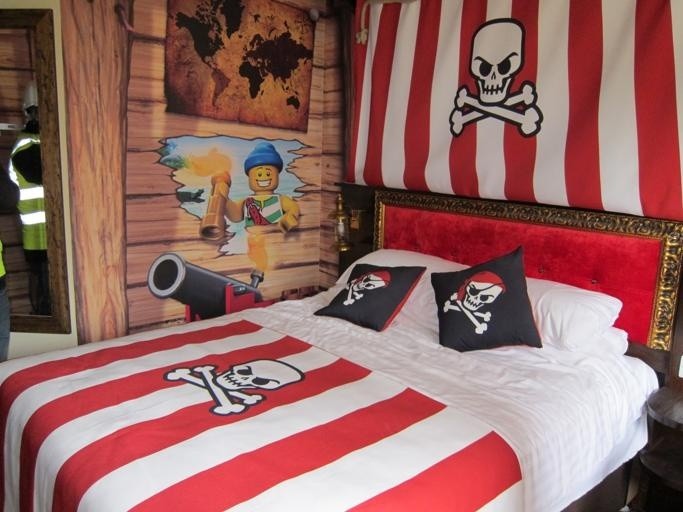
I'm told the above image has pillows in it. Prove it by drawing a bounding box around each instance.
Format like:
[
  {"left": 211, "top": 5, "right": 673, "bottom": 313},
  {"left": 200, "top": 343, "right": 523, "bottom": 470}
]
[
  {"left": 431, "top": 246, "right": 542, "bottom": 352},
  {"left": 573, "top": 328, "right": 631, "bottom": 394},
  {"left": 525, "top": 277, "right": 623, "bottom": 370},
  {"left": 328, "top": 249, "right": 471, "bottom": 336},
  {"left": 313, "top": 263, "right": 427, "bottom": 331}
]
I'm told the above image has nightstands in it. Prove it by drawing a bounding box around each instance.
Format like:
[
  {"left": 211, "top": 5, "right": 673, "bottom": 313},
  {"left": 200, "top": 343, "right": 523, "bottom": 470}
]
[{"left": 639, "top": 377, "right": 683, "bottom": 512}]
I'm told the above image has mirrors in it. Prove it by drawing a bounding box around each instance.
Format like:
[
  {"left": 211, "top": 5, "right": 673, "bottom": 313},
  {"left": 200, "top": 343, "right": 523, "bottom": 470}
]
[{"left": 0, "top": 8, "right": 72, "bottom": 335}]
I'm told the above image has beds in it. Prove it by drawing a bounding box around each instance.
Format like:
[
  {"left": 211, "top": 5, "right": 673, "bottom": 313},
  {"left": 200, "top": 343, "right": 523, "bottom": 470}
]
[{"left": 0, "top": 182, "right": 683, "bottom": 512}]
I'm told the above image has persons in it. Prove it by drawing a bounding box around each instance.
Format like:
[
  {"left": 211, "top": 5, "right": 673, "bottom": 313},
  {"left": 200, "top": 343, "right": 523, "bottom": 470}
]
[
  {"left": 7, "top": 81, "right": 51, "bottom": 315},
  {"left": 0, "top": 240, "right": 11, "bottom": 363}
]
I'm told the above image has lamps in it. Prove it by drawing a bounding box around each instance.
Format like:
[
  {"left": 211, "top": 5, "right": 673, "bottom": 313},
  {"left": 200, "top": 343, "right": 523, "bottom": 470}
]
[{"left": 327, "top": 193, "right": 361, "bottom": 252}]
